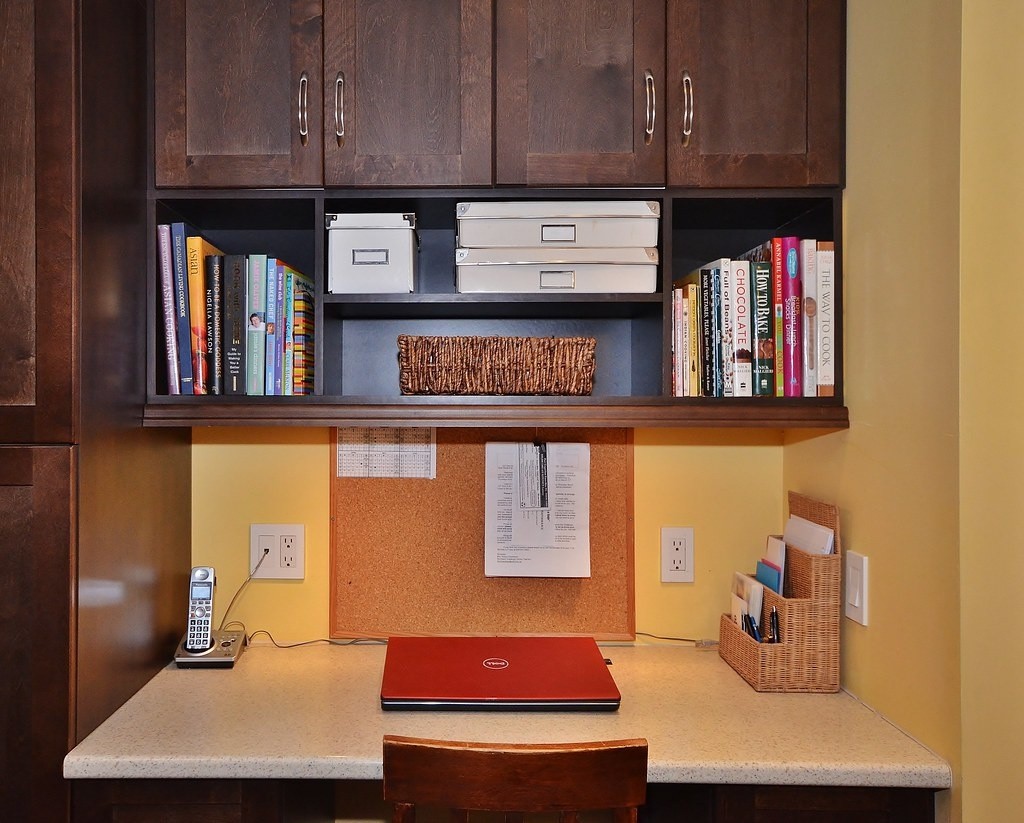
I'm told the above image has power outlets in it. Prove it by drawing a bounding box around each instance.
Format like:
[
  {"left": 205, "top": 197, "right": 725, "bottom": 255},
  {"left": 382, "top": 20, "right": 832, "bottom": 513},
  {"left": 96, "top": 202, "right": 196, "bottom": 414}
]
[
  {"left": 251, "top": 524, "right": 305, "bottom": 579},
  {"left": 661, "top": 526, "right": 694, "bottom": 582}
]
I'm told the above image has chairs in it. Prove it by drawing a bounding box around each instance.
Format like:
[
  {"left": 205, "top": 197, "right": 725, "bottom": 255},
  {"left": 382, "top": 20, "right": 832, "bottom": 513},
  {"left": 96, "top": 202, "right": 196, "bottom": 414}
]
[{"left": 381, "top": 735, "right": 648, "bottom": 823}]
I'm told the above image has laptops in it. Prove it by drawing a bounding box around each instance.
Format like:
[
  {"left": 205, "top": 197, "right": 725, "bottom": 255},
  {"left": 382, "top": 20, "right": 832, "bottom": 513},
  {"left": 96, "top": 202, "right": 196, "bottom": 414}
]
[{"left": 380, "top": 635, "right": 621, "bottom": 712}]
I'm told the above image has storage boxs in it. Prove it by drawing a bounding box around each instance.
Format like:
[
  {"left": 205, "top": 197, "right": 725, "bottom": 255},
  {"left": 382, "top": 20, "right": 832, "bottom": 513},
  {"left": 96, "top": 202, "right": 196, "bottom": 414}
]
[
  {"left": 455, "top": 202, "right": 660, "bottom": 248},
  {"left": 325, "top": 212, "right": 422, "bottom": 294},
  {"left": 455, "top": 247, "right": 659, "bottom": 293}
]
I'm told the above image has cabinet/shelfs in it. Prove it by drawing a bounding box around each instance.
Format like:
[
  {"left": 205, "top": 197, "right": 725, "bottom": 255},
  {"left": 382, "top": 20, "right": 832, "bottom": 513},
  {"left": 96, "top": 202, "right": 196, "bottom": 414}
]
[
  {"left": 642, "top": 783, "right": 935, "bottom": 823},
  {"left": 0, "top": 0, "right": 192, "bottom": 823},
  {"left": 143, "top": 0, "right": 852, "bottom": 428},
  {"left": 71, "top": 779, "right": 335, "bottom": 823}
]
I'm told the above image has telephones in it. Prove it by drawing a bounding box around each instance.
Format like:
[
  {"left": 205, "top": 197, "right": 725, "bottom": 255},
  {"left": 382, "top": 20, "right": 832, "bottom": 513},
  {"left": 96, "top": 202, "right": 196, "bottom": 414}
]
[{"left": 174, "top": 565, "right": 249, "bottom": 670}]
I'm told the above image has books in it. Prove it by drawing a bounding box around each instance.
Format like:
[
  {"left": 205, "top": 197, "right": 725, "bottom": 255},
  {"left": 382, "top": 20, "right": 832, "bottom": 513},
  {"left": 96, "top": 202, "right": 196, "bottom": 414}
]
[
  {"left": 156, "top": 223, "right": 315, "bottom": 396},
  {"left": 783, "top": 515, "right": 834, "bottom": 555},
  {"left": 755, "top": 536, "right": 785, "bottom": 595},
  {"left": 731, "top": 572, "right": 763, "bottom": 634},
  {"left": 673, "top": 237, "right": 835, "bottom": 399}
]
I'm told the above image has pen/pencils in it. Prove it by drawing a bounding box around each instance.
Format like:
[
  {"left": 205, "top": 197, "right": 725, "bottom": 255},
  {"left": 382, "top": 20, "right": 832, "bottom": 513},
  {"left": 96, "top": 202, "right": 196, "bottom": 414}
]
[{"left": 746, "top": 606, "right": 780, "bottom": 644}]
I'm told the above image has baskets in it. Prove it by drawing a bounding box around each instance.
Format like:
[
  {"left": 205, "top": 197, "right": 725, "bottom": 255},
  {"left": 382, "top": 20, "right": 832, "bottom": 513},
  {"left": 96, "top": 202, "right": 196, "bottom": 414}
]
[
  {"left": 719, "top": 490, "right": 841, "bottom": 693},
  {"left": 398, "top": 334, "right": 597, "bottom": 395}
]
